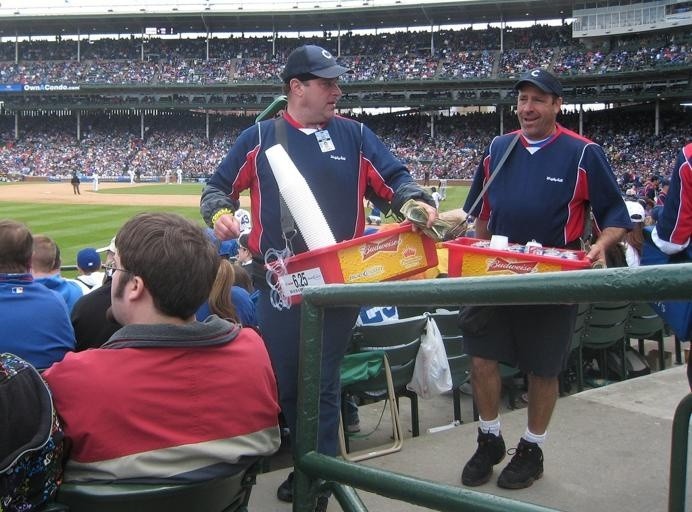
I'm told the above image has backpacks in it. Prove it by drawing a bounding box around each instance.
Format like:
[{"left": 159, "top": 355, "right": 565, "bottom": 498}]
[{"left": 608, "top": 344, "right": 651, "bottom": 380}]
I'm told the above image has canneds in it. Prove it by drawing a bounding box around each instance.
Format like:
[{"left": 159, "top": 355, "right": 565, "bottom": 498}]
[{"left": 471, "top": 239, "right": 578, "bottom": 261}]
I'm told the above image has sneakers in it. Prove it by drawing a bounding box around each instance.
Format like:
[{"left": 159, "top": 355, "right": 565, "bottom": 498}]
[
  {"left": 277, "top": 466, "right": 296, "bottom": 502},
  {"left": 461, "top": 425, "right": 506, "bottom": 487},
  {"left": 497, "top": 437, "right": 544, "bottom": 489}
]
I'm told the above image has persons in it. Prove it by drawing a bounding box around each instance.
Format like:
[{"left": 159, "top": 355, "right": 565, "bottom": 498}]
[
  {"left": 128, "top": 167, "right": 136, "bottom": 184},
  {"left": 200, "top": 45, "right": 439, "bottom": 502},
  {"left": 164, "top": 168, "right": 172, "bottom": 184},
  {"left": 652, "top": 138, "right": 691, "bottom": 391},
  {"left": 71, "top": 176, "right": 81, "bottom": 194},
  {"left": 176, "top": 166, "right": 183, "bottom": 184},
  {"left": 439, "top": 176, "right": 447, "bottom": 200},
  {"left": 135, "top": 170, "right": 140, "bottom": 183},
  {"left": 431, "top": 187, "right": 441, "bottom": 216},
  {"left": 0, "top": 22, "right": 691, "bottom": 179},
  {"left": 342, "top": 201, "right": 691, "bottom": 431},
  {"left": 423, "top": 172, "right": 429, "bottom": 186},
  {"left": 1, "top": 211, "right": 283, "bottom": 511},
  {"left": 92, "top": 172, "right": 99, "bottom": 192},
  {"left": 618, "top": 171, "right": 669, "bottom": 205},
  {"left": 461, "top": 66, "right": 633, "bottom": 491}
]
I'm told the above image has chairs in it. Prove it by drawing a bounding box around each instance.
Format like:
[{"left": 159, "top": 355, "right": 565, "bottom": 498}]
[
  {"left": 426, "top": 312, "right": 480, "bottom": 424},
  {"left": 55, "top": 457, "right": 263, "bottom": 512},
  {"left": 340, "top": 312, "right": 426, "bottom": 454},
  {"left": 569, "top": 301, "right": 682, "bottom": 394}
]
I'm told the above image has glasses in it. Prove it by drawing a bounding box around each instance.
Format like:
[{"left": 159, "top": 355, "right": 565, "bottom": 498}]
[{"left": 102, "top": 259, "right": 139, "bottom": 275}]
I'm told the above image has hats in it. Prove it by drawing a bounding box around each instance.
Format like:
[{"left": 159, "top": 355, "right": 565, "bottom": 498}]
[
  {"left": 513, "top": 68, "right": 563, "bottom": 97},
  {"left": 76, "top": 236, "right": 118, "bottom": 270},
  {"left": 280, "top": 43, "right": 355, "bottom": 79},
  {"left": 625, "top": 200, "right": 648, "bottom": 226}
]
